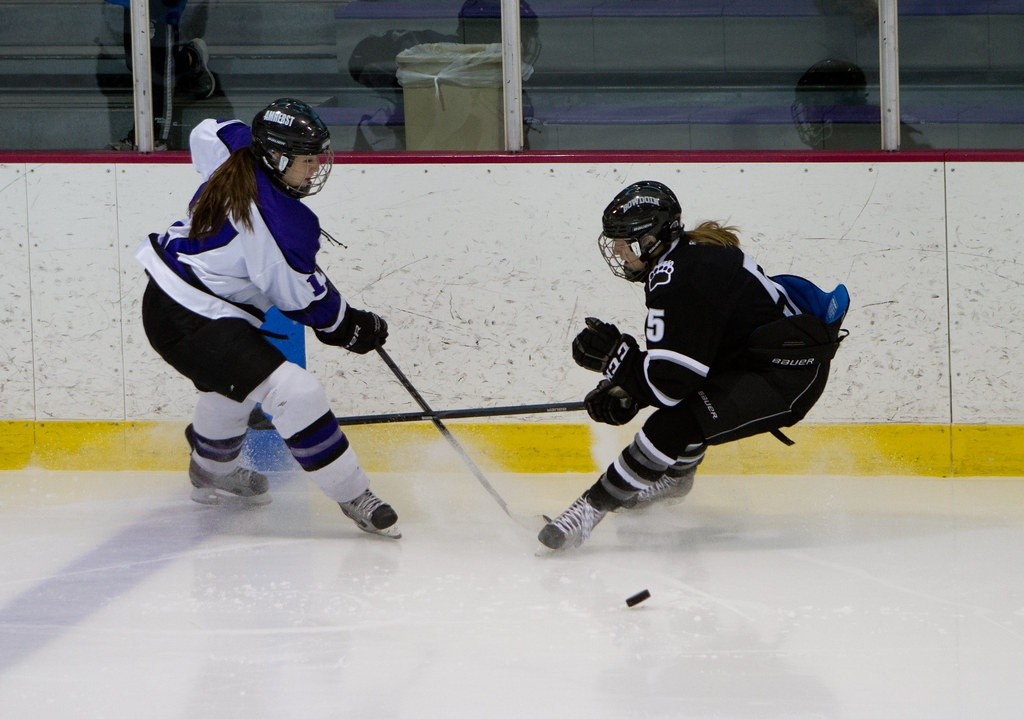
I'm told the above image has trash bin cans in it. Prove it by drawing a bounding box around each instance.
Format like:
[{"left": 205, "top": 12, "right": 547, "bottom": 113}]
[{"left": 395, "top": 41, "right": 509, "bottom": 152}]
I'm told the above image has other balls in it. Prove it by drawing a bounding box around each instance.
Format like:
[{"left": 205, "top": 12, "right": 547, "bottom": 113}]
[{"left": 626, "top": 589, "right": 652, "bottom": 608}]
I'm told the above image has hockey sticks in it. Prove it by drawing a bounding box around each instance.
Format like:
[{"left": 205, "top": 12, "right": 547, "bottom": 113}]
[
  {"left": 375, "top": 347, "right": 554, "bottom": 531},
  {"left": 248, "top": 400, "right": 591, "bottom": 431}
]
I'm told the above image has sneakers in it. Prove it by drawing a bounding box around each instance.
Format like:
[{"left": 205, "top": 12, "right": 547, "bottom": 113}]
[
  {"left": 107, "top": 136, "right": 168, "bottom": 152},
  {"left": 339, "top": 487, "right": 402, "bottom": 540},
  {"left": 184, "top": 37, "right": 215, "bottom": 97},
  {"left": 534, "top": 490, "right": 608, "bottom": 557},
  {"left": 185, "top": 422, "right": 273, "bottom": 507},
  {"left": 610, "top": 468, "right": 697, "bottom": 515}
]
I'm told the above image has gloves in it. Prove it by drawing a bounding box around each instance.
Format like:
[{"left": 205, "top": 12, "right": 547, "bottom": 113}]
[
  {"left": 312, "top": 300, "right": 389, "bottom": 354},
  {"left": 583, "top": 379, "right": 639, "bottom": 426},
  {"left": 572, "top": 317, "right": 640, "bottom": 380}
]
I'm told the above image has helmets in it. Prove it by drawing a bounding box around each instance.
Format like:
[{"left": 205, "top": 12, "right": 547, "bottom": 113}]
[
  {"left": 602, "top": 180, "right": 681, "bottom": 283},
  {"left": 249, "top": 98, "right": 331, "bottom": 199},
  {"left": 795, "top": 58, "right": 867, "bottom": 149},
  {"left": 456, "top": 0, "right": 541, "bottom": 81}
]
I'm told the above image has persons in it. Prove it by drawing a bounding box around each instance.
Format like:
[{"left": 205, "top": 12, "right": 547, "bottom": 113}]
[
  {"left": 141, "top": 98, "right": 398, "bottom": 533},
  {"left": 792, "top": 58, "right": 931, "bottom": 150},
  {"left": 536, "top": 181, "right": 830, "bottom": 552},
  {"left": 350, "top": 0, "right": 540, "bottom": 151},
  {"left": 95, "top": 0, "right": 235, "bottom": 152}
]
